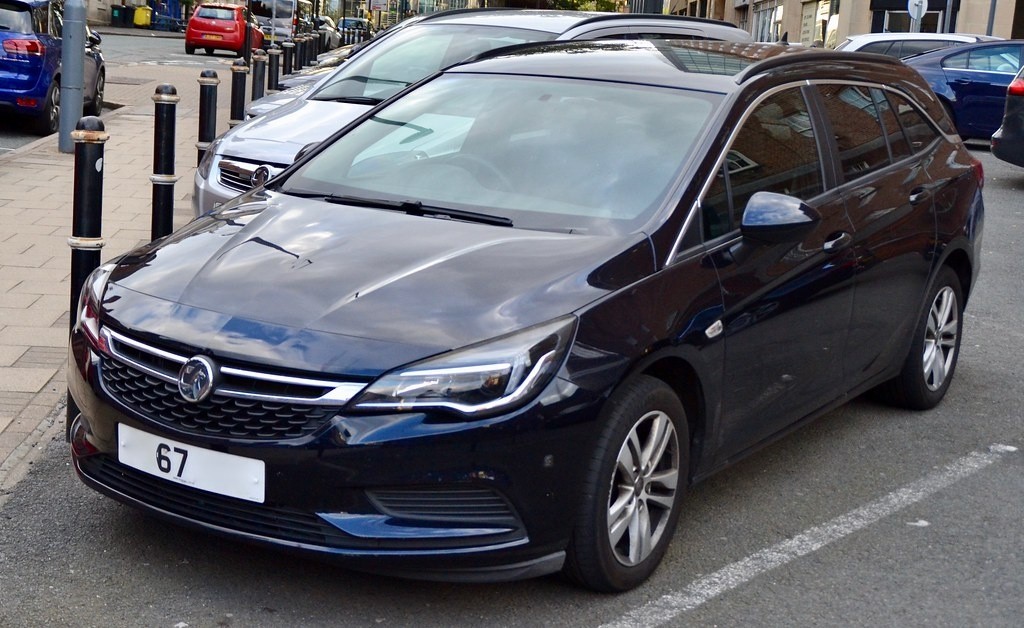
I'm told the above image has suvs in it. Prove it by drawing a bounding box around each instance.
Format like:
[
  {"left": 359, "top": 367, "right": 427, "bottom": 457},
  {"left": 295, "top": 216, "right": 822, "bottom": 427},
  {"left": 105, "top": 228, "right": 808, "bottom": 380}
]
[{"left": 0, "top": 0, "right": 107, "bottom": 135}]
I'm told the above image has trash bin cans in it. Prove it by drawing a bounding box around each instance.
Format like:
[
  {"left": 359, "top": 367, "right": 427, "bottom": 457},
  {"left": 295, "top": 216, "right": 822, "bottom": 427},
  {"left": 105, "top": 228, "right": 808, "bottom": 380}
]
[
  {"left": 111, "top": 4, "right": 126, "bottom": 27},
  {"left": 133, "top": 5, "right": 153, "bottom": 28},
  {"left": 124, "top": 4, "right": 136, "bottom": 27}
]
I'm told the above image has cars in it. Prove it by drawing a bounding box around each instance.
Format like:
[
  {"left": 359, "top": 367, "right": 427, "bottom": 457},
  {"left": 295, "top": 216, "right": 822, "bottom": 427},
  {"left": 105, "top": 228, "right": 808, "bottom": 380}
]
[
  {"left": 183, "top": 3, "right": 267, "bottom": 58},
  {"left": 314, "top": 15, "right": 343, "bottom": 52},
  {"left": 898, "top": 39, "right": 1024, "bottom": 168},
  {"left": 832, "top": 32, "right": 1021, "bottom": 74},
  {"left": 338, "top": 17, "right": 376, "bottom": 41},
  {"left": 195, "top": 7, "right": 754, "bottom": 209},
  {"left": 69, "top": 38, "right": 986, "bottom": 598}
]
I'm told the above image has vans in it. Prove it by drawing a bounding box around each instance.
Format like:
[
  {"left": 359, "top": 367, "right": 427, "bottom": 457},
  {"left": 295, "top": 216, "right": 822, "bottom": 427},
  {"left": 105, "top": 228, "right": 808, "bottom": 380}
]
[{"left": 246, "top": 0, "right": 312, "bottom": 45}]
